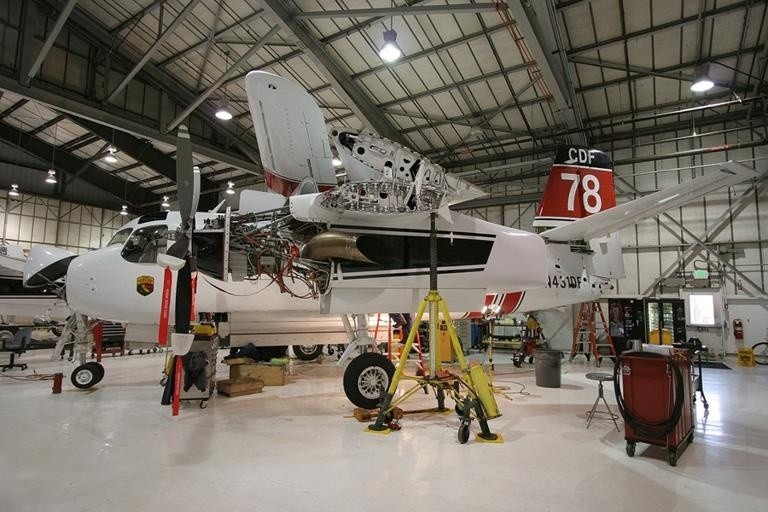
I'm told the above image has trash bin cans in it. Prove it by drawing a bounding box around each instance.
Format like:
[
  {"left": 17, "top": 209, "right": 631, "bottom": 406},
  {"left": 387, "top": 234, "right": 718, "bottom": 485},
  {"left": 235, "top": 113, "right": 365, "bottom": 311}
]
[{"left": 534, "top": 348, "right": 564, "bottom": 388}]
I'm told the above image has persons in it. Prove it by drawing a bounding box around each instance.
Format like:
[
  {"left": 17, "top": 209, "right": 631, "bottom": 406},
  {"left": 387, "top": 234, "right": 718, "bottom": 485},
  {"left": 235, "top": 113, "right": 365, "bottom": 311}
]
[{"left": 610, "top": 304, "right": 624, "bottom": 338}]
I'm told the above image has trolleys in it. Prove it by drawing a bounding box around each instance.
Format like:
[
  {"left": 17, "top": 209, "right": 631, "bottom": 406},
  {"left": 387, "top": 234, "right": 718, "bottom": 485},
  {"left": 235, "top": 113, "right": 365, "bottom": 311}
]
[{"left": 617, "top": 343, "right": 707, "bottom": 466}]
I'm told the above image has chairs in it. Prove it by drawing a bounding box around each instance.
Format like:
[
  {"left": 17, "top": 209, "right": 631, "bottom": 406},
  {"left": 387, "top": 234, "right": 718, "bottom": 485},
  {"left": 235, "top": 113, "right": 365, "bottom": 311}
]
[{"left": 0, "top": 328, "right": 32, "bottom": 372}]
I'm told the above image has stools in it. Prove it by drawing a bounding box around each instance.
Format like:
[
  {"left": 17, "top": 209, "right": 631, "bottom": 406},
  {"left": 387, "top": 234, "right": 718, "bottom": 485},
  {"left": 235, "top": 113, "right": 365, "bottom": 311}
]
[{"left": 584, "top": 372, "right": 620, "bottom": 433}]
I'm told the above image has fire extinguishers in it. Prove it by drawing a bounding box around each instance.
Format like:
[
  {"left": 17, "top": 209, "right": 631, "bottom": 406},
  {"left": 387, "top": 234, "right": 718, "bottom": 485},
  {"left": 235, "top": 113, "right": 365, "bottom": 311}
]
[{"left": 733, "top": 319, "right": 743, "bottom": 339}]
